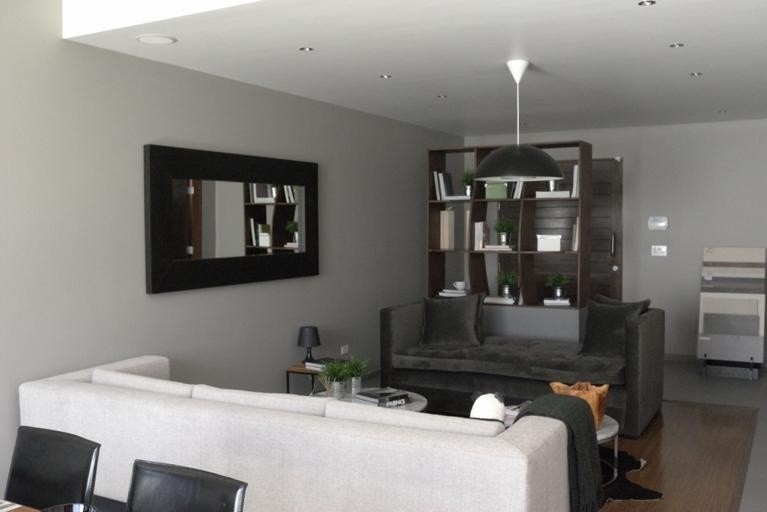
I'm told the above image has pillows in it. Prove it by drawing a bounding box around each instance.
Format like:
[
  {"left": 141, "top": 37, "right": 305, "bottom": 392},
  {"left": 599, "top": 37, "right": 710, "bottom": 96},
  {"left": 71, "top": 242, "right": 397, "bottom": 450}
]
[
  {"left": 419, "top": 288, "right": 488, "bottom": 347},
  {"left": 592, "top": 293, "right": 651, "bottom": 314},
  {"left": 578, "top": 299, "right": 644, "bottom": 361}
]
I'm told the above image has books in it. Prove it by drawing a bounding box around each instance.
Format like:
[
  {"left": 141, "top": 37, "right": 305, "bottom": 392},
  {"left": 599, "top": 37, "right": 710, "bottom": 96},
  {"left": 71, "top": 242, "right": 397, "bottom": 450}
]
[
  {"left": 483, "top": 296, "right": 517, "bottom": 305},
  {"left": 513, "top": 182, "right": 524, "bottom": 199},
  {"left": 249, "top": 183, "right": 275, "bottom": 203},
  {"left": 355, "top": 386, "right": 410, "bottom": 408},
  {"left": 249, "top": 218, "right": 270, "bottom": 246},
  {"left": 507, "top": 400, "right": 532, "bottom": 415},
  {"left": 474, "top": 222, "right": 516, "bottom": 251},
  {"left": 284, "top": 242, "right": 299, "bottom": 248},
  {"left": 439, "top": 288, "right": 468, "bottom": 297},
  {"left": 306, "top": 358, "right": 345, "bottom": 372},
  {"left": 535, "top": 163, "right": 580, "bottom": 199},
  {"left": 283, "top": 184, "right": 295, "bottom": 204},
  {"left": 543, "top": 298, "right": 570, "bottom": 306},
  {"left": 433, "top": 170, "right": 453, "bottom": 200},
  {"left": 572, "top": 217, "right": 580, "bottom": 251}
]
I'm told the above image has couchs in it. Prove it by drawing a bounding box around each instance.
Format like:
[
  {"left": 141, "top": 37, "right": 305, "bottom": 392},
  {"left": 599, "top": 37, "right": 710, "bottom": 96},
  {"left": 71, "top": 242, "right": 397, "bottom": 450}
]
[
  {"left": 379, "top": 298, "right": 665, "bottom": 438},
  {"left": 17, "top": 355, "right": 587, "bottom": 512}
]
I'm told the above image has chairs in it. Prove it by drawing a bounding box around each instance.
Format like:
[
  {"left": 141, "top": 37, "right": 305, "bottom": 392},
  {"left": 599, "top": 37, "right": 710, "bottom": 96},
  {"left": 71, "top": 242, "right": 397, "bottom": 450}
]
[
  {"left": 125, "top": 458, "right": 249, "bottom": 512},
  {"left": 4, "top": 425, "right": 102, "bottom": 512}
]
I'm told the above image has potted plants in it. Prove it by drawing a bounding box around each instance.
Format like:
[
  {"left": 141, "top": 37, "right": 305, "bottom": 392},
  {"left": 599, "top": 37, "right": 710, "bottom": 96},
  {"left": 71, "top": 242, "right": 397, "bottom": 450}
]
[
  {"left": 316, "top": 359, "right": 346, "bottom": 399},
  {"left": 493, "top": 217, "right": 517, "bottom": 246},
  {"left": 344, "top": 356, "right": 370, "bottom": 394},
  {"left": 495, "top": 270, "right": 520, "bottom": 298},
  {"left": 542, "top": 272, "right": 571, "bottom": 299}
]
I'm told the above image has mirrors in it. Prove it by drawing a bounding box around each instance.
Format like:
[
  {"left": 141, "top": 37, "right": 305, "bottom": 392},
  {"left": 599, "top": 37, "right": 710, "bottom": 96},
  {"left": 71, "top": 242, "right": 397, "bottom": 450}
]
[{"left": 143, "top": 143, "right": 320, "bottom": 294}]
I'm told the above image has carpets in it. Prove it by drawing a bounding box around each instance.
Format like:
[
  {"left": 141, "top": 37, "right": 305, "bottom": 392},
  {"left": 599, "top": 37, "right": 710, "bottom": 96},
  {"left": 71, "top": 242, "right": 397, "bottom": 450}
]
[{"left": 599, "top": 447, "right": 664, "bottom": 507}]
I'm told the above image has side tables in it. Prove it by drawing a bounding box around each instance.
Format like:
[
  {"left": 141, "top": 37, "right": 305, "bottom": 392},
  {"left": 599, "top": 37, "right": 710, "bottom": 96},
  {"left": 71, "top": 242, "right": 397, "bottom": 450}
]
[
  {"left": 597, "top": 414, "right": 621, "bottom": 486},
  {"left": 286, "top": 363, "right": 318, "bottom": 395}
]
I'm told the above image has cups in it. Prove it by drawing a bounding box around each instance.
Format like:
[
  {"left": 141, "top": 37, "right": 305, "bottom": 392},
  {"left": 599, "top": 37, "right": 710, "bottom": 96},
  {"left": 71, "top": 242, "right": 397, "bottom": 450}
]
[{"left": 453, "top": 281, "right": 467, "bottom": 290}]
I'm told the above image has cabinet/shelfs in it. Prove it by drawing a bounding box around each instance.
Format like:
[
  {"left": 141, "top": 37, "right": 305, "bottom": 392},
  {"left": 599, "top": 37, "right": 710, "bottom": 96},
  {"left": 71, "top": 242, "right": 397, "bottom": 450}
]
[{"left": 424, "top": 139, "right": 594, "bottom": 308}]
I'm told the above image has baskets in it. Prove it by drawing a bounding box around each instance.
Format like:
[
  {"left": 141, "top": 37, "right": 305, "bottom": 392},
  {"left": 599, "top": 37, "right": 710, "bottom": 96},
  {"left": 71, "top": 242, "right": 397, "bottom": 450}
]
[{"left": 550, "top": 381, "right": 609, "bottom": 430}]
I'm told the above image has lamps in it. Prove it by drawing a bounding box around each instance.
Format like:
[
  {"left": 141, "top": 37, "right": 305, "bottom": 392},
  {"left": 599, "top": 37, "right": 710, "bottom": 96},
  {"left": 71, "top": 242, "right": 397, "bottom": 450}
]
[
  {"left": 297, "top": 326, "right": 321, "bottom": 364},
  {"left": 472, "top": 60, "right": 566, "bottom": 182}
]
